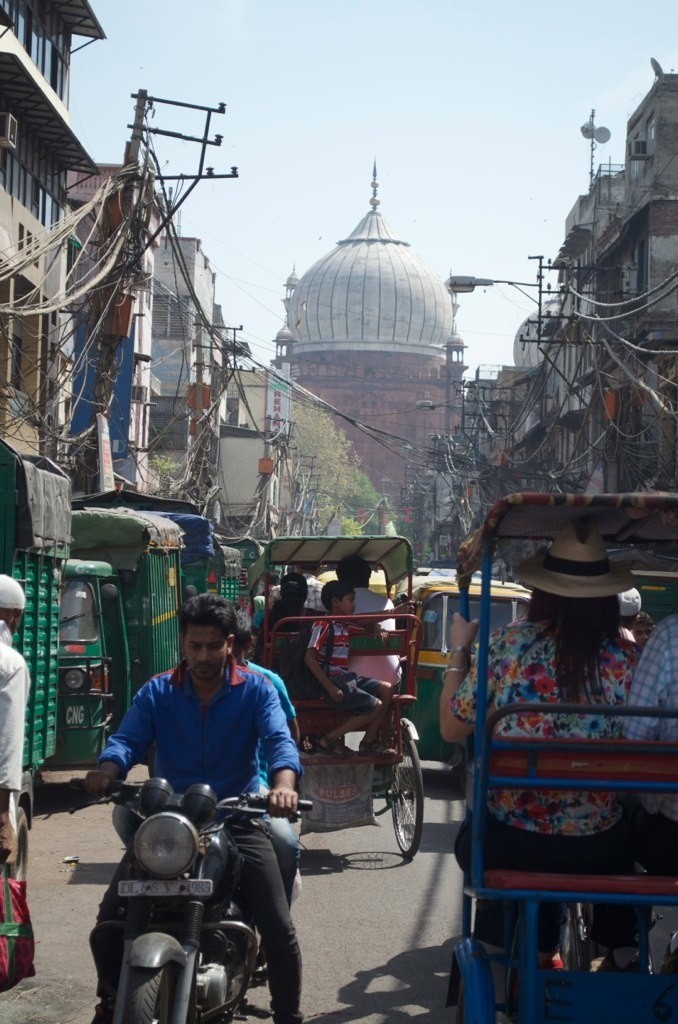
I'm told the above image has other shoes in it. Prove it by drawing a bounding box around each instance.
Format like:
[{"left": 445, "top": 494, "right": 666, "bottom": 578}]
[
  {"left": 91, "top": 997, "right": 115, "bottom": 1024},
  {"left": 543, "top": 959, "right": 564, "bottom": 969},
  {"left": 596, "top": 949, "right": 640, "bottom": 973},
  {"left": 660, "top": 943, "right": 678, "bottom": 972}
]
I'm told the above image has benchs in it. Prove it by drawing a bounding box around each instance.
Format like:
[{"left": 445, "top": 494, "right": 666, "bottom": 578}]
[
  {"left": 462, "top": 702, "right": 678, "bottom": 908},
  {"left": 265, "top": 611, "right": 422, "bottom": 708}
]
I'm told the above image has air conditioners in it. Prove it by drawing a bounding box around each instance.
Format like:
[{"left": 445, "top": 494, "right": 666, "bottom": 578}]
[
  {"left": 0, "top": 112, "right": 17, "bottom": 151},
  {"left": 133, "top": 386, "right": 148, "bottom": 402},
  {"left": 138, "top": 272, "right": 153, "bottom": 291}
]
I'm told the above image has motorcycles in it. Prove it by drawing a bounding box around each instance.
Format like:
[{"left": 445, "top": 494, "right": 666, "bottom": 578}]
[
  {"left": 403, "top": 581, "right": 531, "bottom": 775},
  {"left": 70, "top": 779, "right": 313, "bottom": 1024}
]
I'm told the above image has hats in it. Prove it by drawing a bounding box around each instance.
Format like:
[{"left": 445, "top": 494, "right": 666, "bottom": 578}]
[
  {"left": 618, "top": 588, "right": 642, "bottom": 616},
  {"left": 0, "top": 574, "right": 26, "bottom": 609},
  {"left": 280, "top": 572, "right": 308, "bottom": 600},
  {"left": 519, "top": 528, "right": 634, "bottom": 597}
]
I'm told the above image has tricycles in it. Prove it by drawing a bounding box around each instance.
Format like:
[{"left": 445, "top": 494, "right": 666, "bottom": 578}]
[
  {"left": 248, "top": 533, "right": 427, "bottom": 860},
  {"left": 441, "top": 490, "right": 677, "bottom": 1023}
]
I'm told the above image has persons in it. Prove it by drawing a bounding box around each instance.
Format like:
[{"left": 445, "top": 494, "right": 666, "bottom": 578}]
[{"left": 0, "top": 521, "right": 678, "bottom": 1024}]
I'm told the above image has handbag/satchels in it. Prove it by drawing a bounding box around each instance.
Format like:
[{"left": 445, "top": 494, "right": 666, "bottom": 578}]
[
  {"left": 0, "top": 863, "right": 35, "bottom": 993},
  {"left": 296, "top": 668, "right": 320, "bottom": 701}
]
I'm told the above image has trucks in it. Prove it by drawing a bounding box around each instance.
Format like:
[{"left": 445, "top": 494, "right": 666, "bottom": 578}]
[
  {"left": 0, "top": 439, "right": 73, "bottom": 888},
  {"left": 43, "top": 509, "right": 196, "bottom": 770},
  {"left": 141, "top": 511, "right": 242, "bottom": 608}
]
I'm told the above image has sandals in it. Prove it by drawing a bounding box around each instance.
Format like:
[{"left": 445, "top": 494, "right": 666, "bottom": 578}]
[
  {"left": 359, "top": 738, "right": 398, "bottom": 757},
  {"left": 316, "top": 735, "right": 354, "bottom": 759}
]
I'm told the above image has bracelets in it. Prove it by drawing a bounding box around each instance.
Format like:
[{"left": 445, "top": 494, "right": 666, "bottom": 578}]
[
  {"left": 450, "top": 645, "right": 471, "bottom": 660},
  {"left": 442, "top": 663, "right": 467, "bottom": 681}
]
